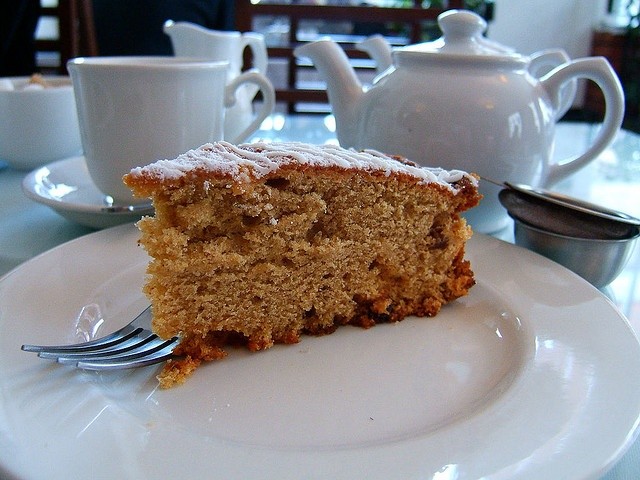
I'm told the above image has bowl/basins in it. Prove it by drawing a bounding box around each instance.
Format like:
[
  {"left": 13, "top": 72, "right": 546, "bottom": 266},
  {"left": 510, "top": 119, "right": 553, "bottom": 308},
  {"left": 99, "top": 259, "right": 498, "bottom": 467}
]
[{"left": 508, "top": 189, "right": 640, "bottom": 290}]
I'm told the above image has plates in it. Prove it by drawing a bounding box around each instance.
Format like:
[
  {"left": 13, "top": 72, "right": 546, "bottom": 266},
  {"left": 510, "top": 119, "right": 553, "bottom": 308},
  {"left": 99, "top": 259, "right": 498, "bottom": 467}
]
[
  {"left": 0, "top": 216, "right": 639, "bottom": 479},
  {"left": 21, "top": 154, "right": 185, "bottom": 228}
]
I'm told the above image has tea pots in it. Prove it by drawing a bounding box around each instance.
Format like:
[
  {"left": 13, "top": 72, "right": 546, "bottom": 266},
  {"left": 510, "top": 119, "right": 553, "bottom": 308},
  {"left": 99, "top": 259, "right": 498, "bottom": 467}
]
[
  {"left": 356, "top": 34, "right": 578, "bottom": 124},
  {"left": 293, "top": 10, "right": 628, "bottom": 236}
]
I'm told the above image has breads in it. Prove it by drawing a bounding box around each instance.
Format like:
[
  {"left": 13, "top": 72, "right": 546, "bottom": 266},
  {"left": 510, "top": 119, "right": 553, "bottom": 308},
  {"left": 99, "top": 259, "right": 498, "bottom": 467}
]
[{"left": 122, "top": 142, "right": 483, "bottom": 388}]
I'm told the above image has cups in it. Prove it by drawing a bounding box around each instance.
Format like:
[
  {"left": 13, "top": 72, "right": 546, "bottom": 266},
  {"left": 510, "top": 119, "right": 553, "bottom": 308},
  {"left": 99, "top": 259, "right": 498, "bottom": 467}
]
[
  {"left": 1, "top": 70, "right": 82, "bottom": 170},
  {"left": 160, "top": 19, "right": 270, "bottom": 144},
  {"left": 66, "top": 56, "right": 276, "bottom": 208}
]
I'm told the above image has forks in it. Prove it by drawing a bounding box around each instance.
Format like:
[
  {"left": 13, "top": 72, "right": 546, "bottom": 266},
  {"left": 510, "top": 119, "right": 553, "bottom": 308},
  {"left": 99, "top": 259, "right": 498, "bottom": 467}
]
[{"left": 20, "top": 288, "right": 192, "bottom": 372}]
